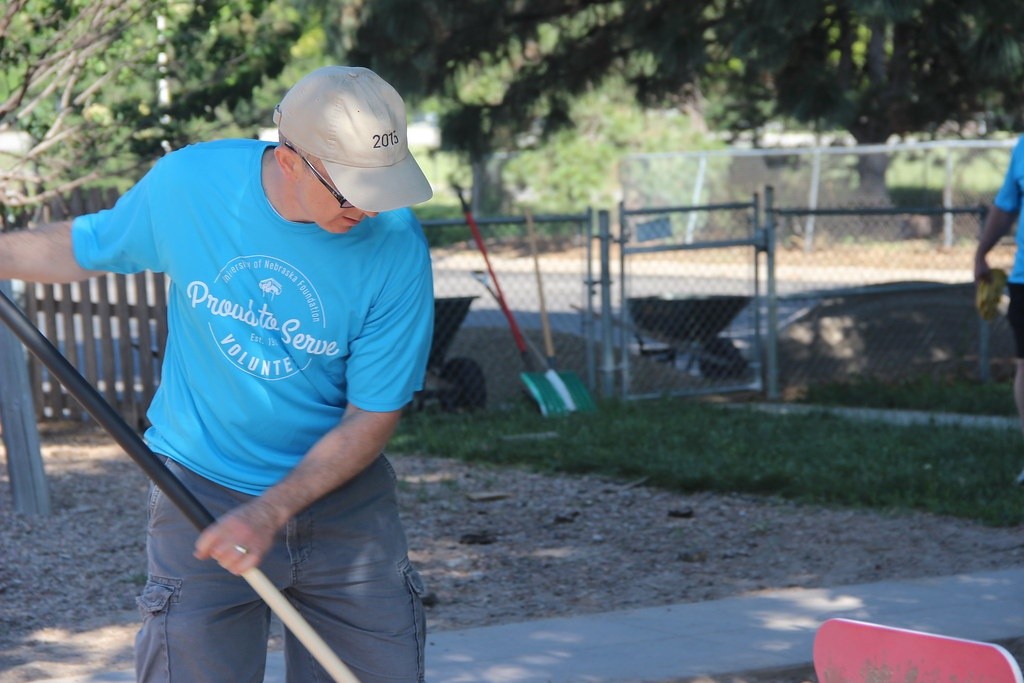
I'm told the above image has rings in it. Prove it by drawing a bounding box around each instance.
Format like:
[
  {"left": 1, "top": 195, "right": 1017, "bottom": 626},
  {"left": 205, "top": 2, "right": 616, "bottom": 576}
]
[{"left": 234, "top": 544, "right": 248, "bottom": 554}]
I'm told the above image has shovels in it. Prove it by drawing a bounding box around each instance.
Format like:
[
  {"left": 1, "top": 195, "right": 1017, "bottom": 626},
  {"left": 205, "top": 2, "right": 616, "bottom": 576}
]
[{"left": 469, "top": 265, "right": 601, "bottom": 421}]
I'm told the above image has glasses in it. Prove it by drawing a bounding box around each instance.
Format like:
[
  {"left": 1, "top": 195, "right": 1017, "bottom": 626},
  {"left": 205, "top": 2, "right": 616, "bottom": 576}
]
[{"left": 284, "top": 141, "right": 355, "bottom": 208}]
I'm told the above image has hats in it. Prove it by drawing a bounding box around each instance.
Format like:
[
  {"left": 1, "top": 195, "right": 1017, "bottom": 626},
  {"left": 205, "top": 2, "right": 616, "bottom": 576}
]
[{"left": 273, "top": 66, "right": 434, "bottom": 212}]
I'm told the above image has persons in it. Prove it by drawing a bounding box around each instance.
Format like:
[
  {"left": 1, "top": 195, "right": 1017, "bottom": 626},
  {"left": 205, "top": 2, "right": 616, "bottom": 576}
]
[
  {"left": 1, "top": 66, "right": 435, "bottom": 683},
  {"left": 976, "top": 131, "right": 1024, "bottom": 487}
]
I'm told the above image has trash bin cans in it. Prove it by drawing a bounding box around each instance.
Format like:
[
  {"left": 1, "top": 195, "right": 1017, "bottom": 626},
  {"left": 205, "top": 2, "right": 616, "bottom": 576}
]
[{"left": 891, "top": 184, "right": 946, "bottom": 238}]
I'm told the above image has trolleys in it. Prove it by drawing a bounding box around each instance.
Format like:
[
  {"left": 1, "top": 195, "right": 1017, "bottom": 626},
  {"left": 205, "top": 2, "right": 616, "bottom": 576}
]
[
  {"left": 570, "top": 291, "right": 755, "bottom": 382},
  {"left": 402, "top": 294, "right": 487, "bottom": 419}
]
